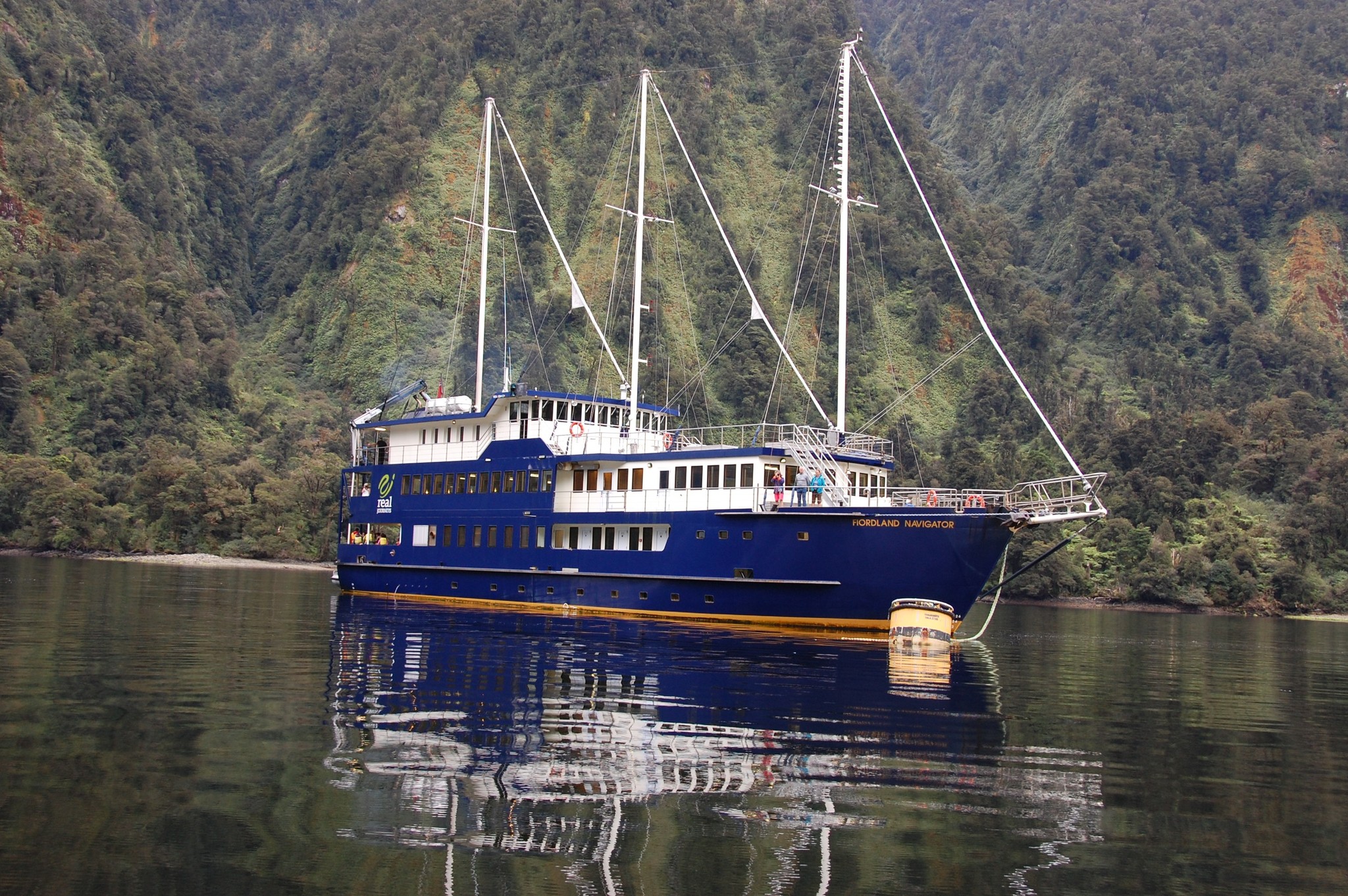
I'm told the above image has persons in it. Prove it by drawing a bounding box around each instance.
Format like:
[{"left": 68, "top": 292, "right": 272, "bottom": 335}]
[
  {"left": 350, "top": 528, "right": 388, "bottom": 545},
  {"left": 810, "top": 470, "right": 825, "bottom": 506},
  {"left": 396, "top": 538, "right": 399, "bottom": 545},
  {"left": 362, "top": 483, "right": 371, "bottom": 496},
  {"left": 795, "top": 467, "right": 811, "bottom": 507},
  {"left": 368, "top": 438, "right": 387, "bottom": 465},
  {"left": 771, "top": 469, "right": 784, "bottom": 506}
]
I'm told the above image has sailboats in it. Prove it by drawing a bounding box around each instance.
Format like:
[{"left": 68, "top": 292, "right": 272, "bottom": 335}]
[{"left": 332, "top": 26, "right": 1108, "bottom": 633}]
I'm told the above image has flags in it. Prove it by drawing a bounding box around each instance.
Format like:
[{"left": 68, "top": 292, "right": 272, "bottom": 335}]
[{"left": 437, "top": 379, "right": 443, "bottom": 398}]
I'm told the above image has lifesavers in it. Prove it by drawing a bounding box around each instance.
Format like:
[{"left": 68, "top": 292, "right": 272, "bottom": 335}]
[
  {"left": 569, "top": 422, "right": 584, "bottom": 437},
  {"left": 927, "top": 490, "right": 937, "bottom": 507},
  {"left": 965, "top": 495, "right": 985, "bottom": 508},
  {"left": 662, "top": 433, "right": 673, "bottom": 449}
]
